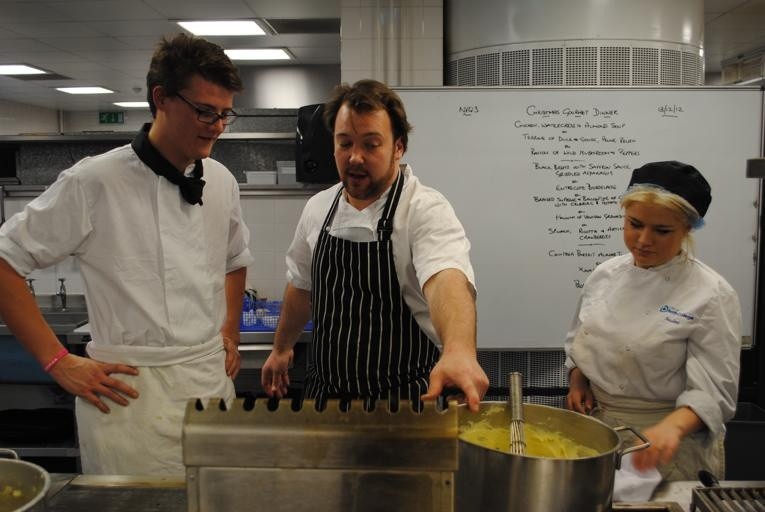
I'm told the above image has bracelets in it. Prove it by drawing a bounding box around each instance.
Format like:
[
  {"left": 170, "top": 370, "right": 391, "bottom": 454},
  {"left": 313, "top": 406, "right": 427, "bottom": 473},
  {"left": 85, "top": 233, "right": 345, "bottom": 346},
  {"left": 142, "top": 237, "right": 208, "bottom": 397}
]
[{"left": 42, "top": 347, "right": 70, "bottom": 376}]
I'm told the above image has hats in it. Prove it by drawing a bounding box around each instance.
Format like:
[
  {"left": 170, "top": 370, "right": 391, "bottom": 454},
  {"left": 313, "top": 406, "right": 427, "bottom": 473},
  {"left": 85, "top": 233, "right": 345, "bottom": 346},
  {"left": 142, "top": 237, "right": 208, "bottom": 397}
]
[{"left": 628, "top": 161, "right": 712, "bottom": 217}]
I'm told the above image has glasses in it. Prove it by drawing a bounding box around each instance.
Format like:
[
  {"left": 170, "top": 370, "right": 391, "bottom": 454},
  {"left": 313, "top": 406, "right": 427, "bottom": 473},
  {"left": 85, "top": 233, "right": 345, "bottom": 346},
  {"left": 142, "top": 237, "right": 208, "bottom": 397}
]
[{"left": 175, "top": 89, "right": 238, "bottom": 126}]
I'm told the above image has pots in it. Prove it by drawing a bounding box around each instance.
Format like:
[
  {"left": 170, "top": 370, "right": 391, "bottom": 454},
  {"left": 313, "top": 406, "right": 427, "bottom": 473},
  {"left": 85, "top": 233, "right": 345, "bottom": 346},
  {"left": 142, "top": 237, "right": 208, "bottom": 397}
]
[
  {"left": 0, "top": 448, "right": 53, "bottom": 512},
  {"left": 454, "top": 400, "right": 651, "bottom": 512}
]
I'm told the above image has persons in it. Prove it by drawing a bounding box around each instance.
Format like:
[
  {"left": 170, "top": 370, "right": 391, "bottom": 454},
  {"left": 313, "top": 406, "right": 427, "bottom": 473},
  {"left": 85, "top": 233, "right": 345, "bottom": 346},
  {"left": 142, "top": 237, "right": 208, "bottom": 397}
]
[
  {"left": 260, "top": 80, "right": 490, "bottom": 414},
  {"left": 0, "top": 36, "right": 252, "bottom": 479},
  {"left": 561, "top": 161, "right": 743, "bottom": 480}
]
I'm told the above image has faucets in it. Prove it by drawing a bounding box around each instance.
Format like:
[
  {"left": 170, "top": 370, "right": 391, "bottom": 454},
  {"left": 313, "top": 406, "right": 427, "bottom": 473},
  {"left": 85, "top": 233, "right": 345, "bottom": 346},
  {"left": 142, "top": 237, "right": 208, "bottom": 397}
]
[
  {"left": 55, "top": 277, "right": 70, "bottom": 311},
  {"left": 26, "top": 278, "right": 37, "bottom": 297}
]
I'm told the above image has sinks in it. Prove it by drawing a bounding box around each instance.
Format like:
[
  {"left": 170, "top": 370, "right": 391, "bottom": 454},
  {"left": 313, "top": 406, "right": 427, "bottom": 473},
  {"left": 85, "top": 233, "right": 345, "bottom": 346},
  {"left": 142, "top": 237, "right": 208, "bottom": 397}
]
[{"left": 0, "top": 312, "right": 88, "bottom": 385}]
